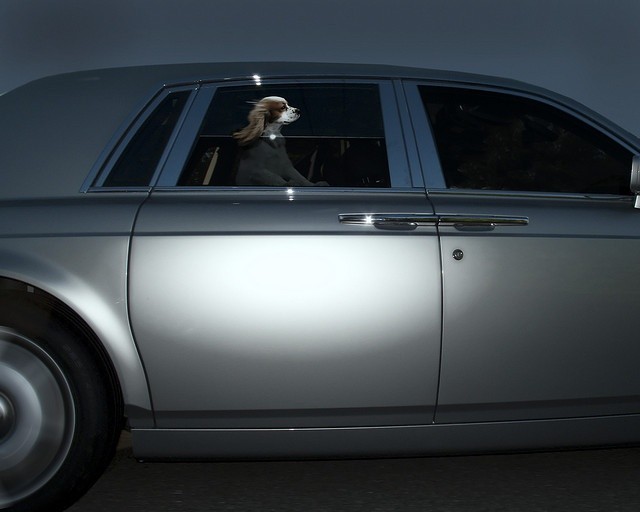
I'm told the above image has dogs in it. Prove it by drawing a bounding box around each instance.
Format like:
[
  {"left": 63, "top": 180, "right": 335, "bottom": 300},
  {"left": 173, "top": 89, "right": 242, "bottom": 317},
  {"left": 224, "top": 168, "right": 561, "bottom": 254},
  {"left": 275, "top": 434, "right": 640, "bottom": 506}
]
[{"left": 233, "top": 94, "right": 326, "bottom": 186}]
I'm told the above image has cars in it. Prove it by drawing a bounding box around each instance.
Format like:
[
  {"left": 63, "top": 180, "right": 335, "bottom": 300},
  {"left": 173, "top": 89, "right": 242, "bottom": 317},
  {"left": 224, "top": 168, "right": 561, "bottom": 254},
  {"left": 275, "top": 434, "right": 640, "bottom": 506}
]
[{"left": 2, "top": 65, "right": 640, "bottom": 505}]
